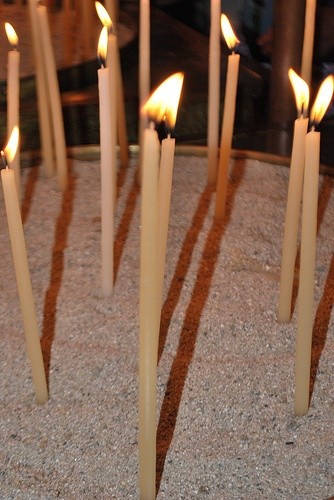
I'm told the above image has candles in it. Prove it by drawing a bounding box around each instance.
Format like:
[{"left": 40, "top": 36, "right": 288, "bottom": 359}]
[{"left": 1, "top": 0, "right": 334, "bottom": 500}]
